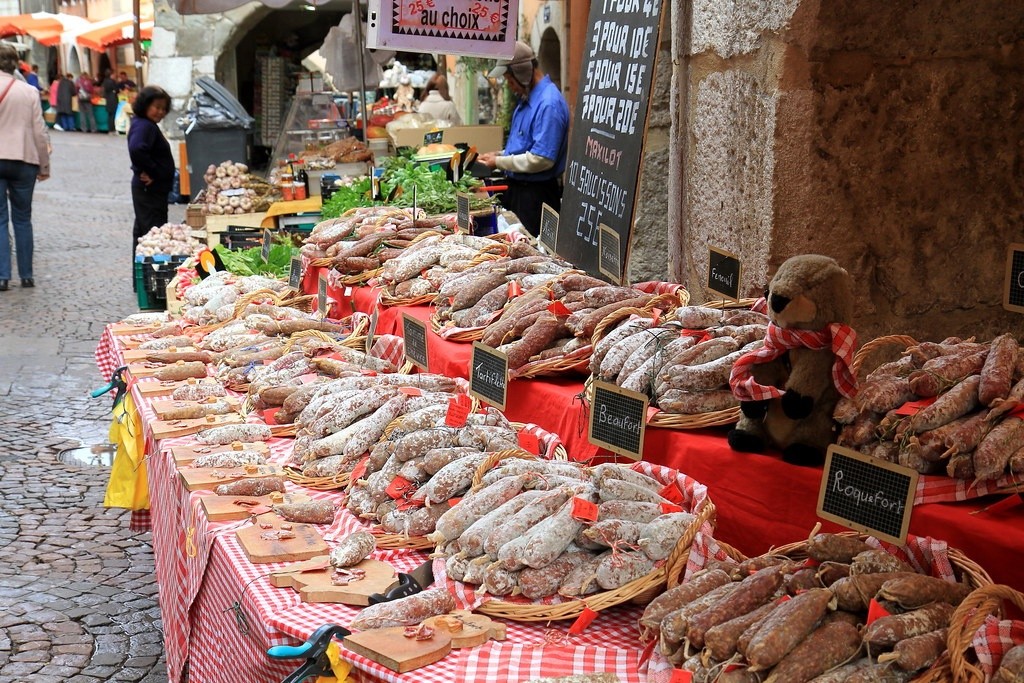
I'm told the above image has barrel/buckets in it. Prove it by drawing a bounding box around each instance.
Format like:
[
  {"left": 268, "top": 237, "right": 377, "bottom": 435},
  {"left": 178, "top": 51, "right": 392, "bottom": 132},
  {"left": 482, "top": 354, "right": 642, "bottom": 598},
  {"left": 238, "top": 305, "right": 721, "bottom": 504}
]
[
  {"left": 351, "top": 91, "right": 378, "bottom": 120},
  {"left": 333, "top": 92, "right": 349, "bottom": 121}
]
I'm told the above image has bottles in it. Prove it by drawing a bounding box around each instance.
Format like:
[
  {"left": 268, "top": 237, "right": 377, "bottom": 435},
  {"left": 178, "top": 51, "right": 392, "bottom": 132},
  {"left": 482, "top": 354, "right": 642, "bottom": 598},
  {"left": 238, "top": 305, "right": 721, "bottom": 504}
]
[
  {"left": 280, "top": 152, "right": 310, "bottom": 199},
  {"left": 298, "top": 71, "right": 324, "bottom": 92}
]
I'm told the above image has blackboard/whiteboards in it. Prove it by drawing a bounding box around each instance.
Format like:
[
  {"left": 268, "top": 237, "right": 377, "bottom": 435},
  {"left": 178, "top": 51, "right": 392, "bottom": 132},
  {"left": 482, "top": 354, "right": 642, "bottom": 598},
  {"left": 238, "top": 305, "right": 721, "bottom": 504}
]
[{"left": 557, "top": 0, "right": 668, "bottom": 284}]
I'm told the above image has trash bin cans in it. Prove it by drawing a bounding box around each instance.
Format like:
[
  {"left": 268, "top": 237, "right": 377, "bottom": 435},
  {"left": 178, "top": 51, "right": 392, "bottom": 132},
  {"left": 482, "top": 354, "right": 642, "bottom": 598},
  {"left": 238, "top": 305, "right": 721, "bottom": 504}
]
[{"left": 178, "top": 72, "right": 256, "bottom": 203}]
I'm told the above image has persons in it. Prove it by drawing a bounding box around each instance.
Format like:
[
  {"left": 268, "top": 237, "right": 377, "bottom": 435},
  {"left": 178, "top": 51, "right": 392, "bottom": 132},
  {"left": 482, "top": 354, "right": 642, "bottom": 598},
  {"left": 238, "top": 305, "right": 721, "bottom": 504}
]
[
  {"left": 480, "top": 42, "right": 570, "bottom": 237},
  {"left": 0, "top": 44, "right": 52, "bottom": 290},
  {"left": 128, "top": 86, "right": 176, "bottom": 293},
  {"left": 19, "top": 56, "right": 136, "bottom": 135},
  {"left": 419, "top": 75, "right": 464, "bottom": 126}
]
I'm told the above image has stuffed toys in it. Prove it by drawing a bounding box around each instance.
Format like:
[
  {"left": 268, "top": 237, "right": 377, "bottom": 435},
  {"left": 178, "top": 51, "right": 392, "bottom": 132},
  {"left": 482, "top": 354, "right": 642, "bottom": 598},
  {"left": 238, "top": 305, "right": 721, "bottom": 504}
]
[{"left": 728, "top": 255, "right": 859, "bottom": 465}]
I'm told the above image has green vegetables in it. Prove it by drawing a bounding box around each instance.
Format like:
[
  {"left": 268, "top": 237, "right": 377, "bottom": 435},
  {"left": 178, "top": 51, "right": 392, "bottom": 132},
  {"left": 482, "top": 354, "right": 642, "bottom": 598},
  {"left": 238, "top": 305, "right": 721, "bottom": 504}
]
[{"left": 321, "top": 161, "right": 501, "bottom": 218}]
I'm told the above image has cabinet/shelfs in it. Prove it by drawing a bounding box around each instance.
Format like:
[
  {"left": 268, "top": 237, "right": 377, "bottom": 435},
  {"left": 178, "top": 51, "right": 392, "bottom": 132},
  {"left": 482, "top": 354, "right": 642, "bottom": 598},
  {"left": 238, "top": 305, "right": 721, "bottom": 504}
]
[{"left": 265, "top": 93, "right": 367, "bottom": 199}]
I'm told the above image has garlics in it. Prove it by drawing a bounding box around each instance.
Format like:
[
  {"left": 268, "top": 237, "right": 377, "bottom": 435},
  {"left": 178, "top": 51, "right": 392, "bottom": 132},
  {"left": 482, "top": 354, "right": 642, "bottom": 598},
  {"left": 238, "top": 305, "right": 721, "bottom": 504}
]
[
  {"left": 203, "top": 160, "right": 253, "bottom": 215},
  {"left": 137, "top": 223, "right": 206, "bottom": 258}
]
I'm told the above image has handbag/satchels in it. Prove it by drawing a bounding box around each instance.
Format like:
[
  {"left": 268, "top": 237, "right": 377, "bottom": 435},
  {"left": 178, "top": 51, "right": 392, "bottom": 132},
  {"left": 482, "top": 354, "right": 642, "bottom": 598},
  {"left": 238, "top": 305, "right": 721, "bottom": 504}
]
[
  {"left": 44, "top": 106, "right": 58, "bottom": 124},
  {"left": 78, "top": 88, "right": 93, "bottom": 102}
]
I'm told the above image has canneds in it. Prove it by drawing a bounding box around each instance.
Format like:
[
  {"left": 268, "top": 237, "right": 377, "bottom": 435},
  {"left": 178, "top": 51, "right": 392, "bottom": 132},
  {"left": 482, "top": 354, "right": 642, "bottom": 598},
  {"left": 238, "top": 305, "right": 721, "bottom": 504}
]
[
  {"left": 281, "top": 174, "right": 293, "bottom": 184},
  {"left": 282, "top": 185, "right": 293, "bottom": 200},
  {"left": 295, "top": 182, "right": 305, "bottom": 199}
]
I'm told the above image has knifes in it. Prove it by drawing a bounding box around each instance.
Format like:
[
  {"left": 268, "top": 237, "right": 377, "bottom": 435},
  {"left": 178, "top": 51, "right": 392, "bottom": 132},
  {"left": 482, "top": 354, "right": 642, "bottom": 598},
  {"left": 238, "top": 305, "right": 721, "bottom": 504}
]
[{"left": 367, "top": 559, "right": 433, "bottom": 604}]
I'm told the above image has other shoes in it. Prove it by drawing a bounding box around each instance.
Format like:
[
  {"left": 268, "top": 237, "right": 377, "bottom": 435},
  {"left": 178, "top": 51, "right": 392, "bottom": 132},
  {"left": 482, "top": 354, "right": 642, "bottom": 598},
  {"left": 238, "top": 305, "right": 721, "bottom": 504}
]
[
  {"left": 21, "top": 278, "right": 35, "bottom": 288},
  {"left": 0, "top": 280, "right": 9, "bottom": 290},
  {"left": 52, "top": 122, "right": 64, "bottom": 132}
]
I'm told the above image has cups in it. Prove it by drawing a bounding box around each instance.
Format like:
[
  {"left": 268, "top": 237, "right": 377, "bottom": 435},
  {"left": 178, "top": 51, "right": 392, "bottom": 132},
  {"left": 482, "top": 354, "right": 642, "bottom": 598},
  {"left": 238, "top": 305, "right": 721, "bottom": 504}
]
[{"left": 282, "top": 181, "right": 307, "bottom": 202}]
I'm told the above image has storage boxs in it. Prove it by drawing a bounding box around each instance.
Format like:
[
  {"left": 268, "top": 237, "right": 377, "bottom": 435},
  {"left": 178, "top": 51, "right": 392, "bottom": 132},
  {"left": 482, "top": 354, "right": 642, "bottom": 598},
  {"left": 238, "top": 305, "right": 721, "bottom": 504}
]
[
  {"left": 133, "top": 248, "right": 191, "bottom": 310},
  {"left": 388, "top": 126, "right": 504, "bottom": 155},
  {"left": 186, "top": 206, "right": 207, "bottom": 230}
]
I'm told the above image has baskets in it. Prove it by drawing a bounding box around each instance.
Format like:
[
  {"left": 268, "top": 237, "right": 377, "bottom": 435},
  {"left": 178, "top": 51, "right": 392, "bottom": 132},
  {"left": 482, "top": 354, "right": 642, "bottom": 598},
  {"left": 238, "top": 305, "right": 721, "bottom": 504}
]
[{"left": 187, "top": 208, "right": 207, "bottom": 228}]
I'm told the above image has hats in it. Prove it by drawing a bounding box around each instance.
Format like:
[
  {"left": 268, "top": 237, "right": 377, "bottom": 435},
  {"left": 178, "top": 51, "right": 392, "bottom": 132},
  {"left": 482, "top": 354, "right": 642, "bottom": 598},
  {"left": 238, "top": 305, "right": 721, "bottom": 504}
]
[{"left": 486, "top": 42, "right": 535, "bottom": 87}]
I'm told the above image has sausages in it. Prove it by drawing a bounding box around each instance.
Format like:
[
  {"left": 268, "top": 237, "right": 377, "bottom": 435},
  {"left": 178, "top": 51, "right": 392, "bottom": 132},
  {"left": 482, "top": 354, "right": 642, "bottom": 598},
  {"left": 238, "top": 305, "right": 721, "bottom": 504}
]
[{"left": 154, "top": 209, "right": 1024, "bottom": 683}]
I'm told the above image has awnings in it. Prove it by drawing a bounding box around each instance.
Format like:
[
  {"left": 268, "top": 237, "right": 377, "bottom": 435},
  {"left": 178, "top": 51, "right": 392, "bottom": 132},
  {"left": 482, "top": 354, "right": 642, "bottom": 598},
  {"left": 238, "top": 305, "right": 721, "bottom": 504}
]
[{"left": 0, "top": 0, "right": 155, "bottom": 52}]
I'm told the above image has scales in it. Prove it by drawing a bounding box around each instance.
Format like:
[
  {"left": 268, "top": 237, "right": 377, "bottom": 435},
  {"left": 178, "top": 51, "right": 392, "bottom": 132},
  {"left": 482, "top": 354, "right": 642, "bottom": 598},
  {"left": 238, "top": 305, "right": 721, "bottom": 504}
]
[{"left": 411, "top": 150, "right": 492, "bottom": 177}]
